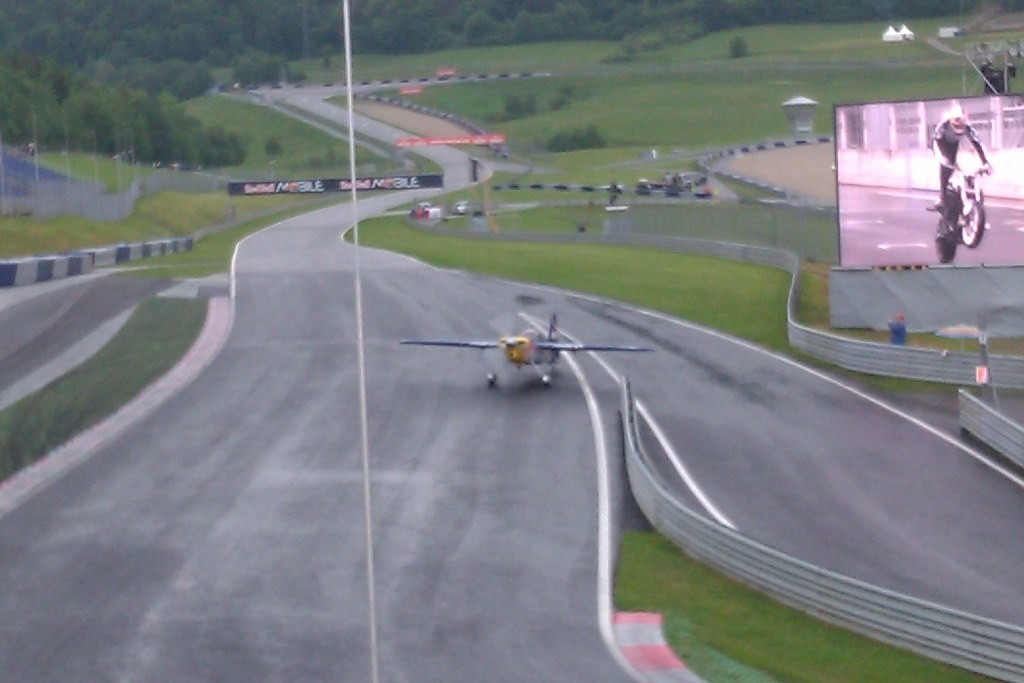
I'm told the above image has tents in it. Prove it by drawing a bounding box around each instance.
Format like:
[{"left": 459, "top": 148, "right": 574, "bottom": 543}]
[{"left": 883, "top": 24, "right": 914, "bottom": 43}]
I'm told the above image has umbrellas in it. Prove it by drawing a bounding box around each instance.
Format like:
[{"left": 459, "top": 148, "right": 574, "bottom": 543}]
[{"left": 935, "top": 324, "right": 981, "bottom": 351}]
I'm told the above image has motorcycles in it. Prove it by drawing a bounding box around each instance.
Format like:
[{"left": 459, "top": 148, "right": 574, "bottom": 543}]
[{"left": 925, "top": 160, "right": 990, "bottom": 265}]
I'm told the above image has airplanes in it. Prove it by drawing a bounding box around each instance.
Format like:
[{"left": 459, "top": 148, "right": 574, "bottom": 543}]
[{"left": 397, "top": 307, "right": 655, "bottom": 384}]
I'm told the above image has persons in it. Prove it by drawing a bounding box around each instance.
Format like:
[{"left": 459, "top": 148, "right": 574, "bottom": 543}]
[
  {"left": 933, "top": 104, "right": 993, "bottom": 204},
  {"left": 888, "top": 313, "right": 906, "bottom": 346},
  {"left": 664, "top": 172, "right": 672, "bottom": 186}
]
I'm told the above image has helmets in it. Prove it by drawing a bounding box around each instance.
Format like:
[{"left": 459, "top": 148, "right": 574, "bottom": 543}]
[{"left": 943, "top": 103, "right": 963, "bottom": 119}]
